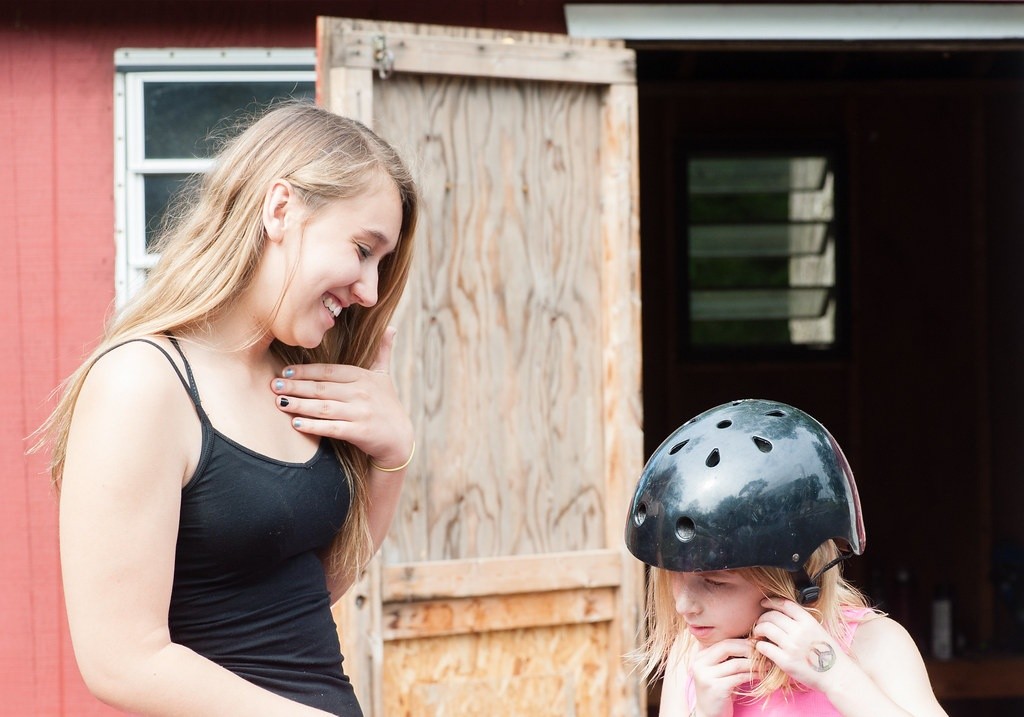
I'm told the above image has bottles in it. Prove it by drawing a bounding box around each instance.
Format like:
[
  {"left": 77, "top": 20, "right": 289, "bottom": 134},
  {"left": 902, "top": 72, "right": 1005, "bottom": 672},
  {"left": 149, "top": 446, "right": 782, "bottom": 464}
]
[
  {"left": 893, "top": 566, "right": 917, "bottom": 643},
  {"left": 930, "top": 584, "right": 954, "bottom": 662}
]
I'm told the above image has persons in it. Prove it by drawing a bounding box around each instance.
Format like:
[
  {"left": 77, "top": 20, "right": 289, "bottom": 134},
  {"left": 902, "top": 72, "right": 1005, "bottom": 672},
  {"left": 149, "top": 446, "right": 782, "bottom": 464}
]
[
  {"left": 24, "top": 92, "right": 420, "bottom": 717},
  {"left": 623, "top": 397, "right": 951, "bottom": 716}
]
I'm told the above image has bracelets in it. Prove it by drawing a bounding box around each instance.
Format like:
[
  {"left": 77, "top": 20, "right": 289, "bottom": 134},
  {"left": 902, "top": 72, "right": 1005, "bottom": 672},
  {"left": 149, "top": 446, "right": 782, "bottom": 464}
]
[{"left": 368, "top": 439, "right": 416, "bottom": 472}]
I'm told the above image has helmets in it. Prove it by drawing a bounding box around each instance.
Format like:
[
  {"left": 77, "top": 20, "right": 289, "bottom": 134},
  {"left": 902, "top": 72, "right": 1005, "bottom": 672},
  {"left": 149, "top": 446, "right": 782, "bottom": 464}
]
[{"left": 625, "top": 399, "right": 866, "bottom": 573}]
